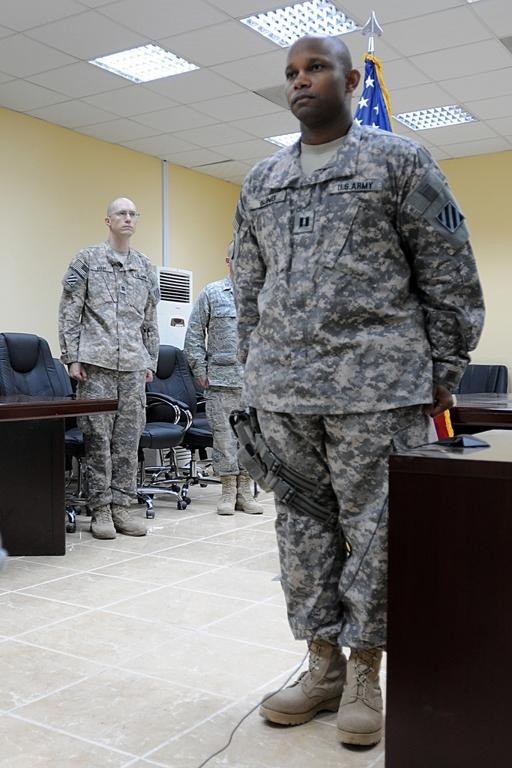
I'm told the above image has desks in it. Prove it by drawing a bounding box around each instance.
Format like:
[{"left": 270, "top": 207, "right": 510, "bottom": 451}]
[
  {"left": 1, "top": 391, "right": 120, "bottom": 559},
  {"left": 439, "top": 391, "right": 511, "bottom": 440},
  {"left": 382, "top": 429, "right": 512, "bottom": 768}
]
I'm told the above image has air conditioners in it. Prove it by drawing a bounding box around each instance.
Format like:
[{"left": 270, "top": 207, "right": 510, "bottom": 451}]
[{"left": 137, "top": 263, "right": 196, "bottom": 473}]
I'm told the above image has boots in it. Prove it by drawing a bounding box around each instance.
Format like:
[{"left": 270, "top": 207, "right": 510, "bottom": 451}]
[
  {"left": 110, "top": 503, "right": 149, "bottom": 537},
  {"left": 216, "top": 475, "right": 238, "bottom": 517},
  {"left": 257, "top": 631, "right": 350, "bottom": 727},
  {"left": 336, "top": 640, "right": 385, "bottom": 749},
  {"left": 89, "top": 504, "right": 117, "bottom": 540},
  {"left": 234, "top": 474, "right": 265, "bottom": 515}
]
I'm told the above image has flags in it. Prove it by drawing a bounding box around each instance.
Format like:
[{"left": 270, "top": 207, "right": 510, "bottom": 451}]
[{"left": 354, "top": 52, "right": 457, "bottom": 444}]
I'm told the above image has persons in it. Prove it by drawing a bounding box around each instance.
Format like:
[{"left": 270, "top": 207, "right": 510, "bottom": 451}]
[
  {"left": 58, "top": 199, "right": 163, "bottom": 540},
  {"left": 224, "top": 36, "right": 486, "bottom": 746},
  {"left": 183, "top": 238, "right": 263, "bottom": 516}
]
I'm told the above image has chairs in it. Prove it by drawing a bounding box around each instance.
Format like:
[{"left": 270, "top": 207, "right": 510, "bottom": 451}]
[
  {"left": 447, "top": 362, "right": 512, "bottom": 435},
  {"left": 1, "top": 332, "right": 73, "bottom": 397},
  {"left": 136, "top": 344, "right": 223, "bottom": 519},
  {"left": 64, "top": 419, "right": 93, "bottom": 533}
]
